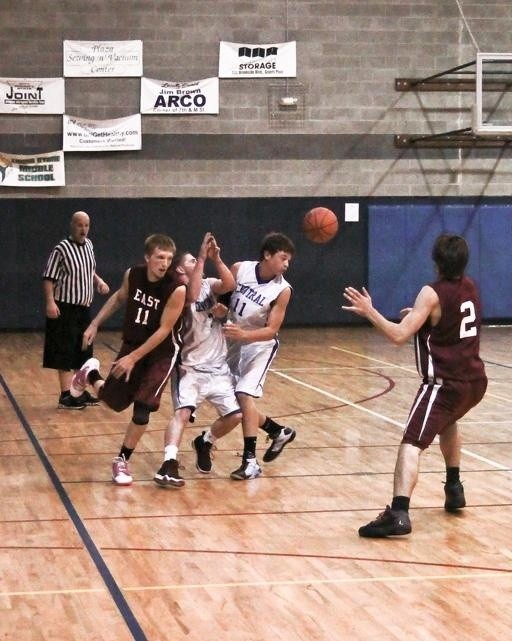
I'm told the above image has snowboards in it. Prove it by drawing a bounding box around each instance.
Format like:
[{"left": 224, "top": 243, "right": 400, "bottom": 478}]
[{"left": 303, "top": 208, "right": 338, "bottom": 243}]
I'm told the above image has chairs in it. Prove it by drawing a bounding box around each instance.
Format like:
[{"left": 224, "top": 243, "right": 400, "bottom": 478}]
[
  {"left": 230, "top": 451, "right": 262, "bottom": 480},
  {"left": 192, "top": 431, "right": 212, "bottom": 473},
  {"left": 358, "top": 505, "right": 411, "bottom": 537},
  {"left": 444, "top": 483, "right": 465, "bottom": 509},
  {"left": 111, "top": 458, "right": 133, "bottom": 485},
  {"left": 69, "top": 358, "right": 100, "bottom": 397},
  {"left": 78, "top": 391, "right": 100, "bottom": 405},
  {"left": 154, "top": 459, "right": 185, "bottom": 487},
  {"left": 263, "top": 426, "right": 296, "bottom": 462},
  {"left": 59, "top": 396, "right": 86, "bottom": 409}
]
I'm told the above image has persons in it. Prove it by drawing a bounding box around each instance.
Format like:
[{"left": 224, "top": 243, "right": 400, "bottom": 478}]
[
  {"left": 335, "top": 235, "right": 488, "bottom": 542},
  {"left": 153, "top": 231, "right": 242, "bottom": 488},
  {"left": 40, "top": 208, "right": 113, "bottom": 414},
  {"left": 215, "top": 227, "right": 300, "bottom": 483},
  {"left": 69, "top": 232, "right": 189, "bottom": 487}
]
